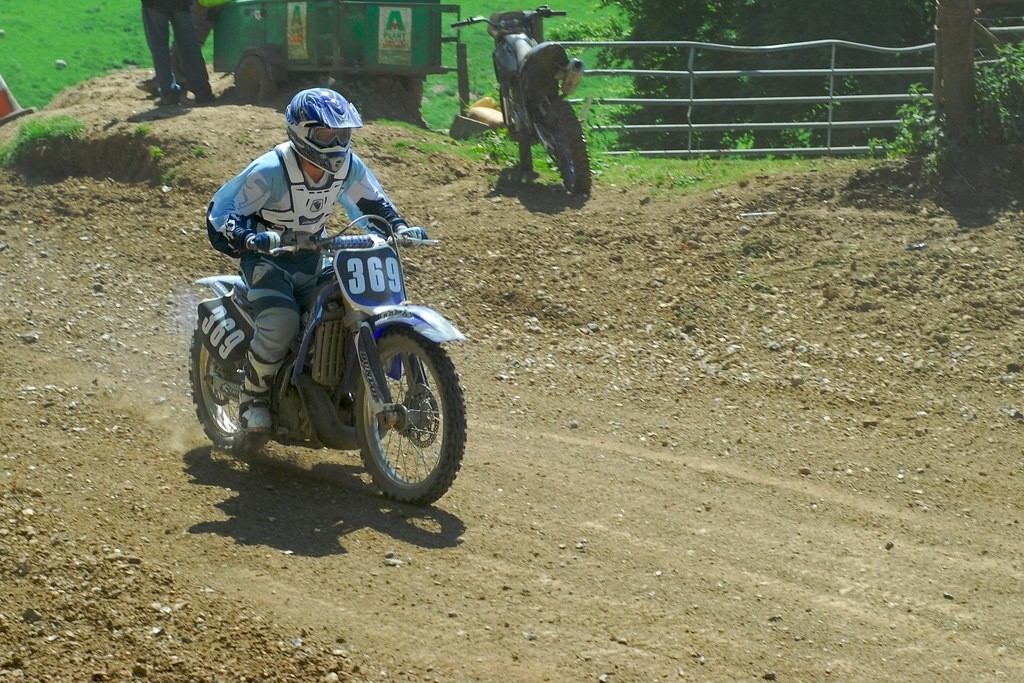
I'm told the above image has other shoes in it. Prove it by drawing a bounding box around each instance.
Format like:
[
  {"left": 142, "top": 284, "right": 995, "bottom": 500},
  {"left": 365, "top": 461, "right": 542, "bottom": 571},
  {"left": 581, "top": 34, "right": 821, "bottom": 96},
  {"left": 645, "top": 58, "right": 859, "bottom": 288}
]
[
  {"left": 136, "top": 76, "right": 162, "bottom": 97},
  {"left": 155, "top": 92, "right": 179, "bottom": 105},
  {"left": 193, "top": 92, "right": 216, "bottom": 103}
]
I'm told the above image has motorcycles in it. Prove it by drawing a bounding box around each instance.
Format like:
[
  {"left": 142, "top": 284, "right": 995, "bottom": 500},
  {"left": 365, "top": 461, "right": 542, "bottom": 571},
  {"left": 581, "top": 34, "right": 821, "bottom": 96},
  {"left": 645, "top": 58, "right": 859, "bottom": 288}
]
[
  {"left": 451, "top": 5, "right": 595, "bottom": 197},
  {"left": 187, "top": 215, "right": 470, "bottom": 505}
]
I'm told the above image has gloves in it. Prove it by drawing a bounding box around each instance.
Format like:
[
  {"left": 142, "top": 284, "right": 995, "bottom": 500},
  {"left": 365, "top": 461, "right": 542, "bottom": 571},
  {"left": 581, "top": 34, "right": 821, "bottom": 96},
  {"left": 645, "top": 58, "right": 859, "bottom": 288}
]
[
  {"left": 398, "top": 227, "right": 429, "bottom": 250},
  {"left": 246, "top": 233, "right": 281, "bottom": 257}
]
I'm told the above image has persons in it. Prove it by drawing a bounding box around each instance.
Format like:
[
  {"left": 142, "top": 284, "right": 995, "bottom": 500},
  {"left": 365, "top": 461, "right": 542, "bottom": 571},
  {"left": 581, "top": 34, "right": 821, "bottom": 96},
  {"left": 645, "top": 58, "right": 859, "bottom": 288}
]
[
  {"left": 136, "top": 0, "right": 221, "bottom": 107},
  {"left": 206, "top": 87, "right": 430, "bottom": 432}
]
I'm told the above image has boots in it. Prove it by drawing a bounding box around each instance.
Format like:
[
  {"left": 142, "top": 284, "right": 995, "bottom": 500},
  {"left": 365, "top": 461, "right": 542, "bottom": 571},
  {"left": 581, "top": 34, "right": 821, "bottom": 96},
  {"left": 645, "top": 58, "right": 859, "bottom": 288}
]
[{"left": 238, "top": 347, "right": 284, "bottom": 433}]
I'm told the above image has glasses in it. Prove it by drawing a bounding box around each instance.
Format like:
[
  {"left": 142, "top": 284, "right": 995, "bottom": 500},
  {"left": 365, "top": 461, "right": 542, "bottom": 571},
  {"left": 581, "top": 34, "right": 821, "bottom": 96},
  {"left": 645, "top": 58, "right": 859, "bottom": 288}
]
[{"left": 316, "top": 131, "right": 345, "bottom": 141}]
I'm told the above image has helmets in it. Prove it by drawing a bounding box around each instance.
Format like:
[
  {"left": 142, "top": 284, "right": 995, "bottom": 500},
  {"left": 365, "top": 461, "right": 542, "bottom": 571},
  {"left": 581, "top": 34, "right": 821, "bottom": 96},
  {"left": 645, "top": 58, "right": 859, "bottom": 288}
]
[{"left": 285, "top": 87, "right": 363, "bottom": 175}]
